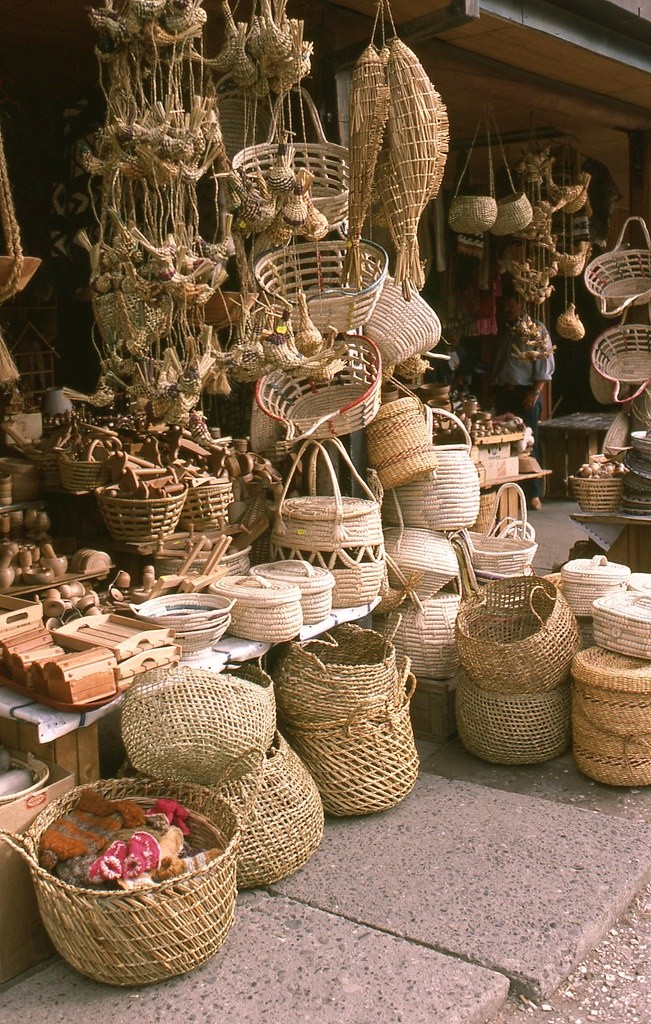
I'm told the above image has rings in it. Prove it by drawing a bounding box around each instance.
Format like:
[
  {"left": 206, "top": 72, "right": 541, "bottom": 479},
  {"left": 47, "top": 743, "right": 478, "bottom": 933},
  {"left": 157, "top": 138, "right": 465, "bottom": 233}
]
[{"left": 529, "top": 402, "right": 532, "bottom": 406}]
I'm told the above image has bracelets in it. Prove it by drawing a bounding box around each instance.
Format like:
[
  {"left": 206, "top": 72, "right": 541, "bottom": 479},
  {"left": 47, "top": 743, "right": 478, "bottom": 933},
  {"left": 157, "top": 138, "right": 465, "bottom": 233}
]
[{"left": 531, "top": 387, "right": 541, "bottom": 395}]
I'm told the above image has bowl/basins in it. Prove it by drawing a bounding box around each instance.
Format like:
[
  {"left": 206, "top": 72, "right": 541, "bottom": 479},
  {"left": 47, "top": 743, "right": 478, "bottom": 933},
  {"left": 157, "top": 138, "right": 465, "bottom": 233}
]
[
  {"left": 0, "top": 473, "right": 13, "bottom": 506},
  {"left": 23, "top": 567, "right": 55, "bottom": 584},
  {"left": 379, "top": 383, "right": 451, "bottom": 412}
]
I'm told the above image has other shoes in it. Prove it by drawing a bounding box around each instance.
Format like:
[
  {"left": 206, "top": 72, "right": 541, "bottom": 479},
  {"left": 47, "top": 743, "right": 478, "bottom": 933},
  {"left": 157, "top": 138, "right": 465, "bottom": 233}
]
[{"left": 529, "top": 496, "right": 542, "bottom": 510}]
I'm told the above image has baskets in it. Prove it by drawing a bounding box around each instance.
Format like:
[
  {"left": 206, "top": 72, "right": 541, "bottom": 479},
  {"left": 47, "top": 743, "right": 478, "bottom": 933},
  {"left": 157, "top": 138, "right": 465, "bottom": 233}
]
[
  {"left": 604, "top": 408, "right": 651, "bottom": 516},
  {"left": 24, "top": 68, "right": 537, "bottom": 680},
  {"left": 592, "top": 295, "right": 651, "bottom": 403},
  {"left": 582, "top": 215, "right": 651, "bottom": 318},
  {"left": 569, "top": 474, "right": 625, "bottom": 514},
  {"left": 444, "top": 571, "right": 584, "bottom": 766},
  {"left": 0, "top": 611, "right": 418, "bottom": 986}
]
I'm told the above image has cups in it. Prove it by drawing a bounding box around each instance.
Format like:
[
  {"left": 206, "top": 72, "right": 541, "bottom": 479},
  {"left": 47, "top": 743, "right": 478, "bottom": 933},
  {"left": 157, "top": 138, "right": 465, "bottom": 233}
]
[
  {"left": 0, "top": 540, "right": 40, "bottom": 585},
  {"left": 463, "top": 401, "right": 477, "bottom": 418}
]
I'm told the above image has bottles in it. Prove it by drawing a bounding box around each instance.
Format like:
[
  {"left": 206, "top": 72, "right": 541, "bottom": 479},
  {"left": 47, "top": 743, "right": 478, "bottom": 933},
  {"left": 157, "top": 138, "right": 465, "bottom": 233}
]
[
  {"left": 0, "top": 508, "right": 51, "bottom": 543},
  {"left": 233, "top": 439, "right": 247, "bottom": 452},
  {"left": 209, "top": 427, "right": 221, "bottom": 438}
]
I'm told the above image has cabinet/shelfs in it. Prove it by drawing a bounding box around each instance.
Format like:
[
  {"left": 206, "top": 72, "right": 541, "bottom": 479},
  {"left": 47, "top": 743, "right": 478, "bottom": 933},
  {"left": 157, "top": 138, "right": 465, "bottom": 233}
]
[{"left": 0, "top": 499, "right": 117, "bottom": 596}]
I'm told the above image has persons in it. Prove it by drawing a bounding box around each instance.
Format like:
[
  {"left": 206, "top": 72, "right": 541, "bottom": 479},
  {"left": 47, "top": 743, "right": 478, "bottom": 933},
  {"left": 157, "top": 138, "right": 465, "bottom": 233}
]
[
  {"left": 486, "top": 291, "right": 555, "bottom": 510},
  {"left": 426, "top": 326, "right": 478, "bottom": 395}
]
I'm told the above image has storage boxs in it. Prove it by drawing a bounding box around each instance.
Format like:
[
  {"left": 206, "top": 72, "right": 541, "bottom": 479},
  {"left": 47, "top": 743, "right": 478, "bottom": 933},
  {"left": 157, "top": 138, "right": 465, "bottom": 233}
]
[
  {"left": 0, "top": 742, "right": 77, "bottom": 984},
  {"left": 115, "top": 661, "right": 179, "bottom": 692},
  {"left": 405, "top": 675, "right": 456, "bottom": 744},
  {"left": 113, "top": 642, "right": 182, "bottom": 680},
  {"left": 49, "top": 611, "right": 176, "bottom": 663},
  {"left": 480, "top": 443, "right": 519, "bottom": 480}
]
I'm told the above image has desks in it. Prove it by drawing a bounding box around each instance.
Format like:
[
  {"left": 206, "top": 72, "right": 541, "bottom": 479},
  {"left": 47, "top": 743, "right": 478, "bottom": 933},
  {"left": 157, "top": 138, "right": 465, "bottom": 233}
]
[
  {"left": 479, "top": 469, "right": 553, "bottom": 489},
  {"left": 536, "top": 412, "right": 619, "bottom": 498},
  {"left": 570, "top": 511, "right": 651, "bottom": 573},
  {"left": 0, "top": 595, "right": 384, "bottom": 788}
]
